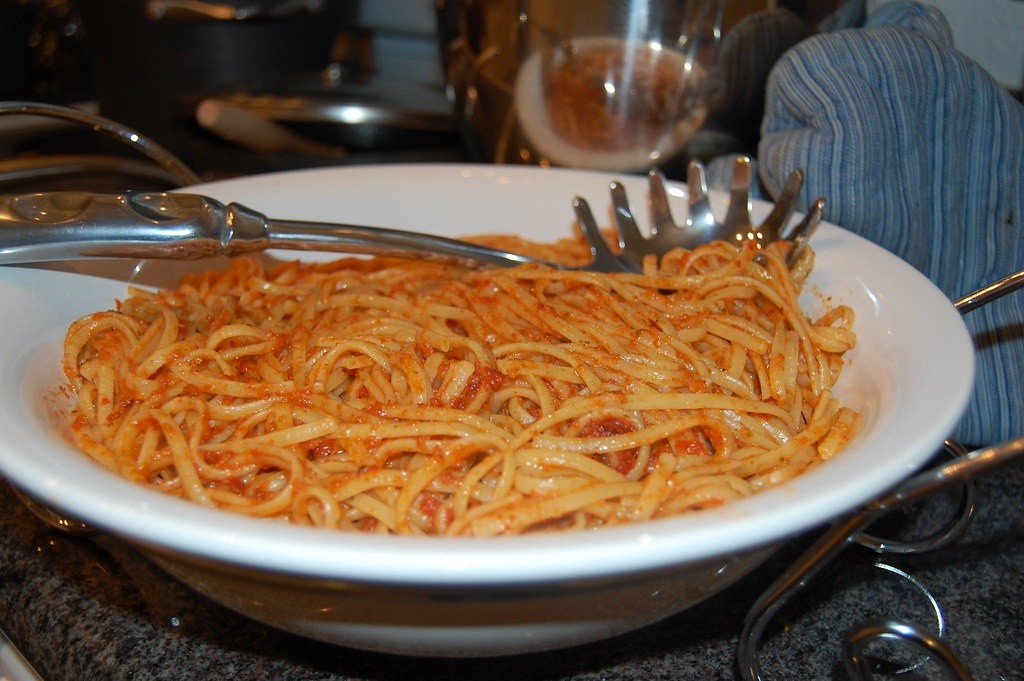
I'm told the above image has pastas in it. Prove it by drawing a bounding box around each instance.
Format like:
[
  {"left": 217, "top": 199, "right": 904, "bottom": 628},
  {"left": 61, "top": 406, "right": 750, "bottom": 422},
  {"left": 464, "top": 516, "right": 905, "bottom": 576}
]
[{"left": 65, "top": 225, "right": 858, "bottom": 545}]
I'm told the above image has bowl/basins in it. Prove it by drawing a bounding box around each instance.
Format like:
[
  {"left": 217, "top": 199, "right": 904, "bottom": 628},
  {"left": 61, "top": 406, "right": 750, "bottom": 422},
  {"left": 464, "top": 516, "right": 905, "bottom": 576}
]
[
  {"left": 1, "top": 160, "right": 978, "bottom": 658},
  {"left": 516, "top": 38, "right": 706, "bottom": 171}
]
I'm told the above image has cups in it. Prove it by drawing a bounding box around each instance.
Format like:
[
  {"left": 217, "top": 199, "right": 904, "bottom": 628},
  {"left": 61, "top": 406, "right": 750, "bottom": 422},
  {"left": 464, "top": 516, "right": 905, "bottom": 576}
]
[{"left": 495, "top": 0, "right": 721, "bottom": 182}]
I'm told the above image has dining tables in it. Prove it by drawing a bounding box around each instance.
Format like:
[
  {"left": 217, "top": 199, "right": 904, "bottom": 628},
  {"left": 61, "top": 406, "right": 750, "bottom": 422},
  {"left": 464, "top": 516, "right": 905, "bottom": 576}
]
[{"left": 0, "top": 453, "right": 1024, "bottom": 681}]
